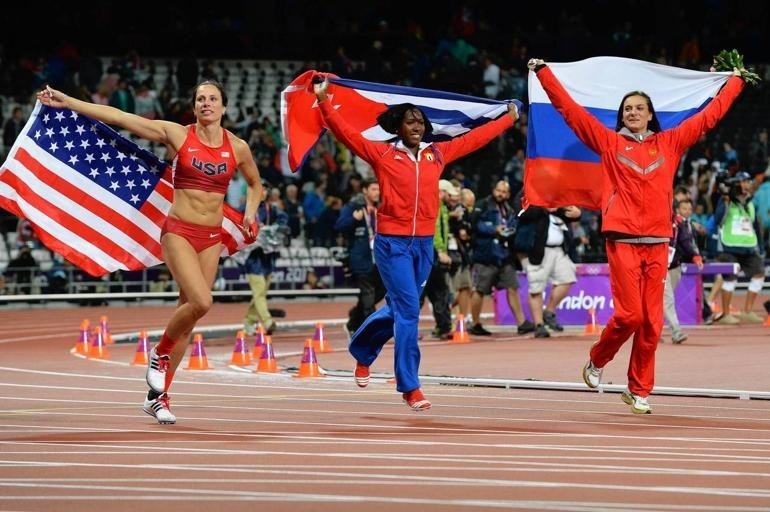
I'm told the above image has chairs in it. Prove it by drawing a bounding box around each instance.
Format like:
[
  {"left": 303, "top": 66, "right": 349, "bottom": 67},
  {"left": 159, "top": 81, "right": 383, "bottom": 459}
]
[{"left": 1, "top": 53, "right": 493, "bottom": 295}]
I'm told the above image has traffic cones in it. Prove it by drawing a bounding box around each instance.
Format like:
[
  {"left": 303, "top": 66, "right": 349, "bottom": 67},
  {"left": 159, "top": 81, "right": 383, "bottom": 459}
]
[
  {"left": 178, "top": 332, "right": 214, "bottom": 371},
  {"left": 451, "top": 311, "right": 476, "bottom": 345},
  {"left": 252, "top": 324, "right": 268, "bottom": 361},
  {"left": 583, "top": 306, "right": 601, "bottom": 335},
  {"left": 87, "top": 325, "right": 109, "bottom": 360},
  {"left": 99, "top": 314, "right": 115, "bottom": 346},
  {"left": 129, "top": 328, "right": 154, "bottom": 367},
  {"left": 253, "top": 334, "right": 282, "bottom": 375},
  {"left": 292, "top": 336, "right": 325, "bottom": 379},
  {"left": 228, "top": 328, "right": 255, "bottom": 367},
  {"left": 76, "top": 316, "right": 93, "bottom": 357},
  {"left": 309, "top": 320, "right": 331, "bottom": 354}
]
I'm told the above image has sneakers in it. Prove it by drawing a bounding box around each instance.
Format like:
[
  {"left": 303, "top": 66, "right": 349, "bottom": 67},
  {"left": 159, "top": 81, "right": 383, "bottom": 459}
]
[
  {"left": 517, "top": 310, "right": 563, "bottom": 339},
  {"left": 582, "top": 359, "right": 603, "bottom": 389},
  {"left": 428, "top": 321, "right": 491, "bottom": 340},
  {"left": 401, "top": 387, "right": 432, "bottom": 412},
  {"left": 141, "top": 388, "right": 177, "bottom": 425},
  {"left": 704, "top": 294, "right": 770, "bottom": 328},
  {"left": 342, "top": 321, "right": 356, "bottom": 344},
  {"left": 620, "top": 387, "right": 651, "bottom": 415},
  {"left": 242, "top": 319, "right": 278, "bottom": 335},
  {"left": 671, "top": 330, "right": 690, "bottom": 345},
  {"left": 144, "top": 342, "right": 171, "bottom": 393},
  {"left": 354, "top": 361, "right": 372, "bottom": 388}
]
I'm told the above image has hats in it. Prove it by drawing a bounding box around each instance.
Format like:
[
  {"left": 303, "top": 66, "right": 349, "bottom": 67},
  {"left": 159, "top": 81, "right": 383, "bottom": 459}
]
[{"left": 437, "top": 179, "right": 457, "bottom": 196}]
